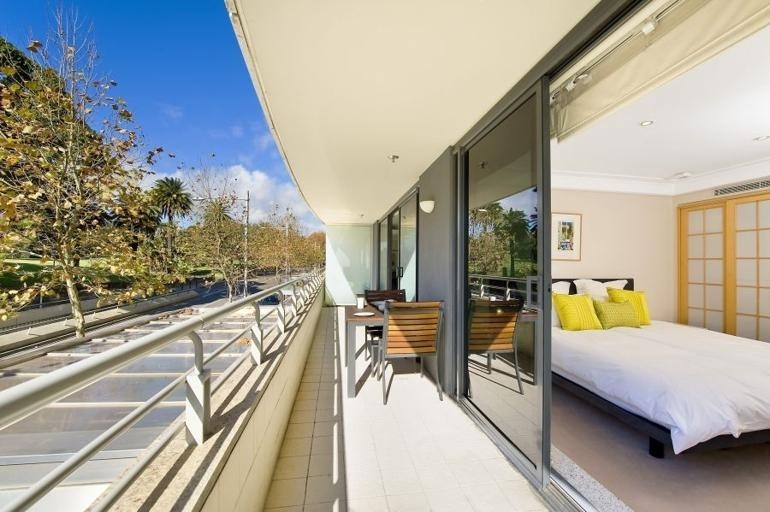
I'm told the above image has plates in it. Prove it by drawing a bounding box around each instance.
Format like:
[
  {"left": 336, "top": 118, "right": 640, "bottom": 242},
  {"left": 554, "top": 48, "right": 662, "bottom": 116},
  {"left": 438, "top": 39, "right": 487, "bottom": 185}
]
[{"left": 353, "top": 312, "right": 375, "bottom": 317}]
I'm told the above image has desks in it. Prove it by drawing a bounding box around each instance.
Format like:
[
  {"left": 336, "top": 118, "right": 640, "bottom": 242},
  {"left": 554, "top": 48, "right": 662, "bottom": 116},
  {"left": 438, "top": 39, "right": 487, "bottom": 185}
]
[
  {"left": 521, "top": 306, "right": 538, "bottom": 385},
  {"left": 344, "top": 304, "right": 384, "bottom": 399}
]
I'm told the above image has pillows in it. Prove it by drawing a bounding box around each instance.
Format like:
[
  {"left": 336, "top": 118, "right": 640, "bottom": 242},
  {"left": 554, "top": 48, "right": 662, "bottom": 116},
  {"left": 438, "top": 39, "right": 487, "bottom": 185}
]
[{"left": 550, "top": 278, "right": 651, "bottom": 332}]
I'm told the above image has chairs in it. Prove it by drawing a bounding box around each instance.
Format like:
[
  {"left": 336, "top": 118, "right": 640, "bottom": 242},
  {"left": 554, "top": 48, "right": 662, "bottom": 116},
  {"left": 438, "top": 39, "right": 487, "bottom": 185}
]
[
  {"left": 469, "top": 297, "right": 526, "bottom": 398},
  {"left": 361, "top": 288, "right": 445, "bottom": 404}
]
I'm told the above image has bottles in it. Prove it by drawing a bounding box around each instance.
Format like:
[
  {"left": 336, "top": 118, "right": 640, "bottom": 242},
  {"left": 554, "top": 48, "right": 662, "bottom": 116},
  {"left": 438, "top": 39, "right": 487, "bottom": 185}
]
[{"left": 505, "top": 288, "right": 511, "bottom": 302}]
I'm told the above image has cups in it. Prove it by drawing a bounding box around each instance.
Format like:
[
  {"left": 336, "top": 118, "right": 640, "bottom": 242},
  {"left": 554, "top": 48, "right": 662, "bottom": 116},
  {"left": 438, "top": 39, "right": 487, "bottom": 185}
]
[
  {"left": 489, "top": 297, "right": 496, "bottom": 302},
  {"left": 357, "top": 297, "right": 364, "bottom": 310}
]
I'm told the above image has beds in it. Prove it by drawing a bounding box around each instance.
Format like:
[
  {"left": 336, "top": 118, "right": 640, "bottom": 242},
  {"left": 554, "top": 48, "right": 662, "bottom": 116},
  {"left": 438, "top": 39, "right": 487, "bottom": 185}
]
[{"left": 551, "top": 277, "right": 770, "bottom": 458}]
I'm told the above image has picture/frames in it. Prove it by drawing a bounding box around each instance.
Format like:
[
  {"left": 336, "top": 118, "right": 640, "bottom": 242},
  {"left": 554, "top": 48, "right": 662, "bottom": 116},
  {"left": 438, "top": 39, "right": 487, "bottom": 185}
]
[{"left": 551, "top": 212, "right": 582, "bottom": 261}]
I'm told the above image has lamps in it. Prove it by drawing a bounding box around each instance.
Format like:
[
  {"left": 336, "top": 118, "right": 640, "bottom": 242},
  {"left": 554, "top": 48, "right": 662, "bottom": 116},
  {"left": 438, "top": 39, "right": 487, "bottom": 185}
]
[{"left": 419, "top": 199, "right": 435, "bottom": 214}]
